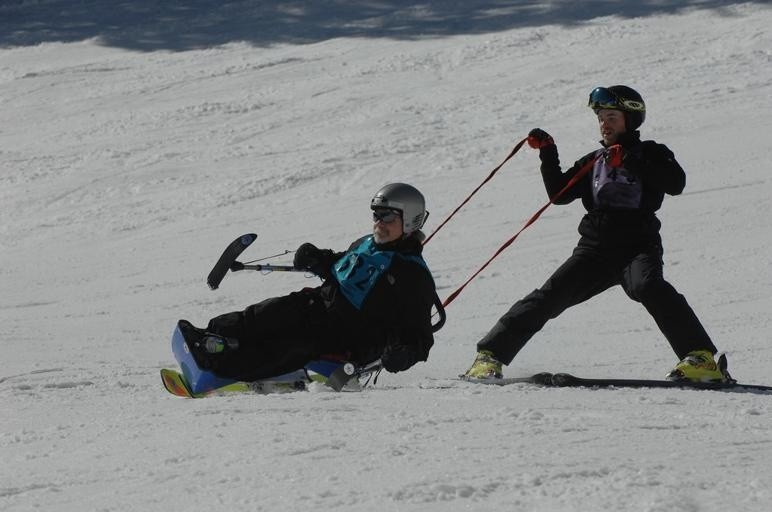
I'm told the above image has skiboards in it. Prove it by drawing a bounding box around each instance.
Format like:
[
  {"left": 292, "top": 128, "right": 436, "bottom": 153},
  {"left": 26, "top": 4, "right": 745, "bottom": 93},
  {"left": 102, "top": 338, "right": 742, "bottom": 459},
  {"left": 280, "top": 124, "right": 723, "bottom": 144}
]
[
  {"left": 161, "top": 369, "right": 331, "bottom": 399},
  {"left": 419, "top": 374, "right": 771, "bottom": 395}
]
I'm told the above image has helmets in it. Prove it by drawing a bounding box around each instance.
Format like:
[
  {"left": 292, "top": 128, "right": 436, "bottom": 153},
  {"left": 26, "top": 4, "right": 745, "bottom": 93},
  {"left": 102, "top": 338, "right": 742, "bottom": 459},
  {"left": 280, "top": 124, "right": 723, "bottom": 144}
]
[
  {"left": 370, "top": 184, "right": 424, "bottom": 239},
  {"left": 591, "top": 86, "right": 645, "bottom": 131}
]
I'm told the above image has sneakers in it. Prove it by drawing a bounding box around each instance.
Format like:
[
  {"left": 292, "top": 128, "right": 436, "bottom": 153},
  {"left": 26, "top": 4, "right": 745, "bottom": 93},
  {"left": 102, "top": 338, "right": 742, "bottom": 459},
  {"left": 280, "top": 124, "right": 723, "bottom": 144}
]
[
  {"left": 467, "top": 348, "right": 503, "bottom": 377},
  {"left": 676, "top": 352, "right": 725, "bottom": 382}
]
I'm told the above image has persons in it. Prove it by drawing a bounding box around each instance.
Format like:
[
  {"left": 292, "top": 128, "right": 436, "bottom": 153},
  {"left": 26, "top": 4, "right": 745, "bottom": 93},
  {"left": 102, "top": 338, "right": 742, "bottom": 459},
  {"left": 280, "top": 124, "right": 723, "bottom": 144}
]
[
  {"left": 145, "top": 179, "right": 437, "bottom": 396},
  {"left": 448, "top": 82, "right": 740, "bottom": 389}
]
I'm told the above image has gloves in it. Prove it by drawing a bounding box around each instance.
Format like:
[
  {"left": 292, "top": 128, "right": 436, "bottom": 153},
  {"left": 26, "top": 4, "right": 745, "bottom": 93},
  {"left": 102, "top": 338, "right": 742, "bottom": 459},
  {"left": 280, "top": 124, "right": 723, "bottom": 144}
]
[
  {"left": 293, "top": 242, "right": 330, "bottom": 269},
  {"left": 529, "top": 128, "right": 554, "bottom": 145},
  {"left": 382, "top": 342, "right": 424, "bottom": 372}
]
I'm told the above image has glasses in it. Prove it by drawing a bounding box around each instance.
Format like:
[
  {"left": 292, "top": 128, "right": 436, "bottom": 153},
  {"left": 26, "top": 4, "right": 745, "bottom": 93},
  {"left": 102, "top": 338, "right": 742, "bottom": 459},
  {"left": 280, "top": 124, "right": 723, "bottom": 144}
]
[
  {"left": 589, "top": 87, "right": 617, "bottom": 109},
  {"left": 372, "top": 212, "right": 402, "bottom": 225}
]
